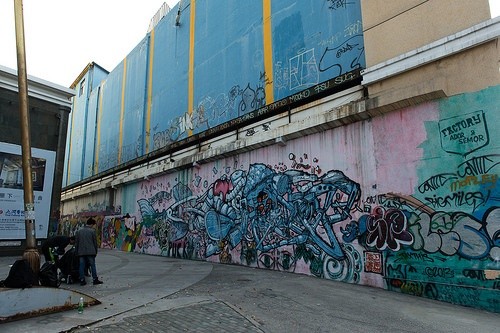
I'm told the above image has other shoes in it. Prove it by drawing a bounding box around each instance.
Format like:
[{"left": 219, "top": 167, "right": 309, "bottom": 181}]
[
  {"left": 92, "top": 276, "right": 103, "bottom": 285},
  {"left": 80, "top": 279, "right": 86, "bottom": 286}
]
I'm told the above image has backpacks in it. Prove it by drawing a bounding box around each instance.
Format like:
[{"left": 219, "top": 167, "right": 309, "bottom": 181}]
[{"left": 39, "top": 261, "right": 60, "bottom": 288}]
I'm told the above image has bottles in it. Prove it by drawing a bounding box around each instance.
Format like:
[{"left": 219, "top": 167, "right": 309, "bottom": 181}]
[{"left": 78, "top": 297, "right": 84, "bottom": 314}]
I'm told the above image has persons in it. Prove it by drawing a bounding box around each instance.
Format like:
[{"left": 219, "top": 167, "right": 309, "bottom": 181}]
[{"left": 5, "top": 218, "right": 104, "bottom": 289}]
